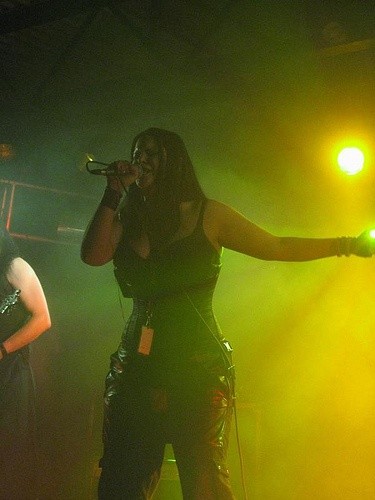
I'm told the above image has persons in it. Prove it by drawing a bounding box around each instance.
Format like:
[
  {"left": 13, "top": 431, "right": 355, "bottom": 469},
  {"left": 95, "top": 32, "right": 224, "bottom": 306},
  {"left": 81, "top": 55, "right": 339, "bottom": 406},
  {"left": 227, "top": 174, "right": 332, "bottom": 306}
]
[
  {"left": 76, "top": 122, "right": 375, "bottom": 500},
  {"left": 1, "top": 199, "right": 54, "bottom": 500}
]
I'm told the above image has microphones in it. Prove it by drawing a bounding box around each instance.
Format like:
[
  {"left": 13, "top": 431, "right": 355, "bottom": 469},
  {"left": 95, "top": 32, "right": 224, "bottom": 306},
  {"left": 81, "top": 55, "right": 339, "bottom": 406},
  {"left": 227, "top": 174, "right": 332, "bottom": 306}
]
[{"left": 92, "top": 165, "right": 144, "bottom": 178}]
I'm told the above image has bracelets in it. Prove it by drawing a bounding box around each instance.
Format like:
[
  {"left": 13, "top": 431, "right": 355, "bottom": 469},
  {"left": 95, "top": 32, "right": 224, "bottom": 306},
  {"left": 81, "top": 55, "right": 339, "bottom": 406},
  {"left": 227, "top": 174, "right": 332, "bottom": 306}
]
[
  {"left": 342, "top": 235, "right": 346, "bottom": 259},
  {"left": 336, "top": 234, "right": 342, "bottom": 259},
  {"left": 347, "top": 236, "right": 353, "bottom": 258}
]
